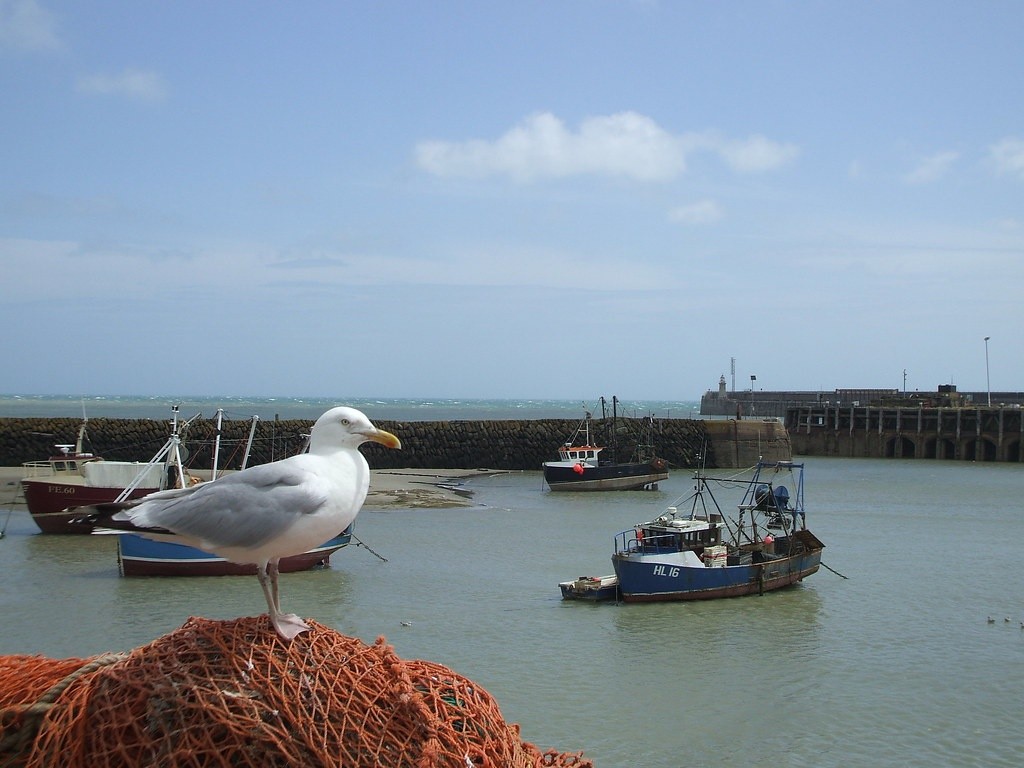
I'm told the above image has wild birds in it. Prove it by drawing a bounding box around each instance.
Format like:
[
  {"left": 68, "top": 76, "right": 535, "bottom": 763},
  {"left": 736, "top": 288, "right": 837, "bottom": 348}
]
[{"left": 31, "top": 406, "right": 401, "bottom": 644}]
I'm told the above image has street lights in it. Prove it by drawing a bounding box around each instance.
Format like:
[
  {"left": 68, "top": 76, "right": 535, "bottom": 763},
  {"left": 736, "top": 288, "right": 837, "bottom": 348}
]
[{"left": 984, "top": 336, "right": 995, "bottom": 406}]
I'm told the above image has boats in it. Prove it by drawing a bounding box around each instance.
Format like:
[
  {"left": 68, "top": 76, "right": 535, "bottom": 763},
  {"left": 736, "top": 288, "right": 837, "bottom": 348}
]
[
  {"left": 541, "top": 394, "right": 672, "bottom": 494},
  {"left": 611, "top": 433, "right": 828, "bottom": 604},
  {"left": 557, "top": 573, "right": 622, "bottom": 603},
  {"left": 19, "top": 404, "right": 313, "bottom": 534},
  {"left": 116, "top": 518, "right": 359, "bottom": 576}
]
